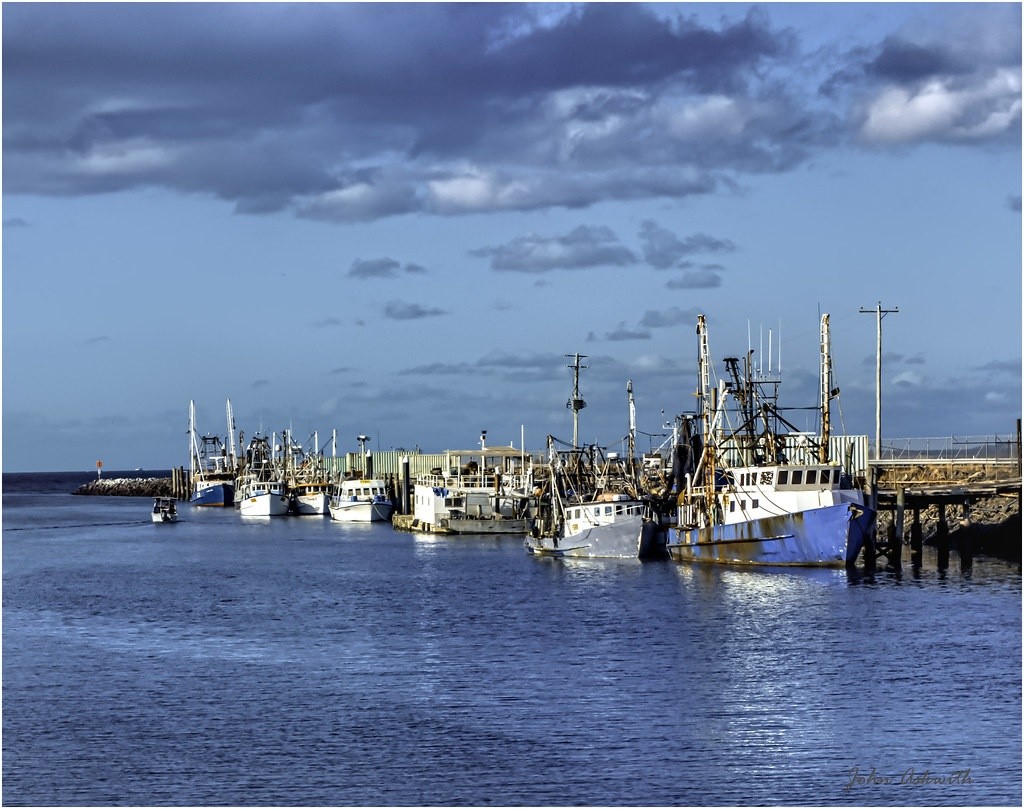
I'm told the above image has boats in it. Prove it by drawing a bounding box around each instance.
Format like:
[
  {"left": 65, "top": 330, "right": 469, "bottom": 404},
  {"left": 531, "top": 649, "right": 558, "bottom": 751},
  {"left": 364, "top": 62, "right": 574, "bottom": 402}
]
[
  {"left": 440, "top": 474, "right": 547, "bottom": 535},
  {"left": 653, "top": 313, "right": 878, "bottom": 568},
  {"left": 327, "top": 466, "right": 394, "bottom": 522},
  {"left": 518, "top": 353, "right": 662, "bottom": 559},
  {"left": 151, "top": 495, "right": 179, "bottom": 523},
  {"left": 231, "top": 418, "right": 332, "bottom": 516}
]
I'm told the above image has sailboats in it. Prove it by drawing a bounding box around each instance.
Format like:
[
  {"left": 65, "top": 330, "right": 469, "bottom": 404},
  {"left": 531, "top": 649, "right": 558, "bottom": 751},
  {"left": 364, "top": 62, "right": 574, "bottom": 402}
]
[{"left": 184, "top": 400, "right": 236, "bottom": 507}]
